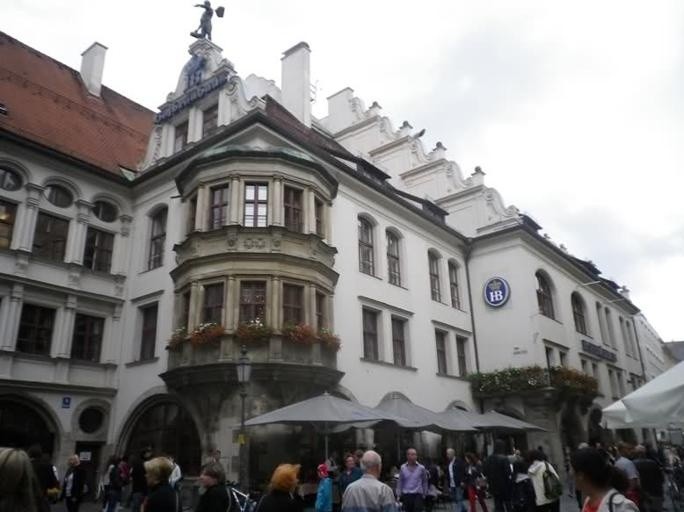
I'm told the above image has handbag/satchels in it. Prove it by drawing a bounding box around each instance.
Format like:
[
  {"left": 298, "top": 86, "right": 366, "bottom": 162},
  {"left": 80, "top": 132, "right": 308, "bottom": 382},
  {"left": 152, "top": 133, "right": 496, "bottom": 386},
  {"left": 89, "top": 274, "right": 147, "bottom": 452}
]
[{"left": 544, "top": 462, "right": 564, "bottom": 498}]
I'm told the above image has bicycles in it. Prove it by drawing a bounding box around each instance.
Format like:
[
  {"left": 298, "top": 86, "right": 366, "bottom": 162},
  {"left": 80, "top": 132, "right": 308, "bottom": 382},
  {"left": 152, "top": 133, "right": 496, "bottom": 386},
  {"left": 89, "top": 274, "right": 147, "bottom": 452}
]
[
  {"left": 661, "top": 466, "right": 680, "bottom": 509},
  {"left": 225, "top": 480, "right": 261, "bottom": 510}
]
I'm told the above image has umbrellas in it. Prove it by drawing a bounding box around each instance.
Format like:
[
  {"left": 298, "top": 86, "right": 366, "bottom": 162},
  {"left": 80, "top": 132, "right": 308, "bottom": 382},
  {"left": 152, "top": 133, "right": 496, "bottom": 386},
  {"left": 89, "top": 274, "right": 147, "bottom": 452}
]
[{"left": 598, "top": 359, "right": 684, "bottom": 430}]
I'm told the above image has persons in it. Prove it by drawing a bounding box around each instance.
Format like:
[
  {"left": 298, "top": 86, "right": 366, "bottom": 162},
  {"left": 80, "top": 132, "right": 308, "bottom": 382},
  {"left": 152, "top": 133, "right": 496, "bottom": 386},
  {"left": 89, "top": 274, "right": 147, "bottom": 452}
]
[{"left": 194, "top": 0, "right": 213, "bottom": 40}]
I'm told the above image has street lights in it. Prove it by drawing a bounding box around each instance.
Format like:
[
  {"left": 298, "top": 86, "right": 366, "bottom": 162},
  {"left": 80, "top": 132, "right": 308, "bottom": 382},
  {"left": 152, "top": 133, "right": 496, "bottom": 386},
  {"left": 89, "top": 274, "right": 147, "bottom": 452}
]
[{"left": 232, "top": 340, "right": 251, "bottom": 492}]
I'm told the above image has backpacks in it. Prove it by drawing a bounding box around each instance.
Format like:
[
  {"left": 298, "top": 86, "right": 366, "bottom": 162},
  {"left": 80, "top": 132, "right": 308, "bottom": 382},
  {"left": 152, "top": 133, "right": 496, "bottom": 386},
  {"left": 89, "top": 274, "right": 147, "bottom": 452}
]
[{"left": 226, "top": 487, "right": 257, "bottom": 512}]
